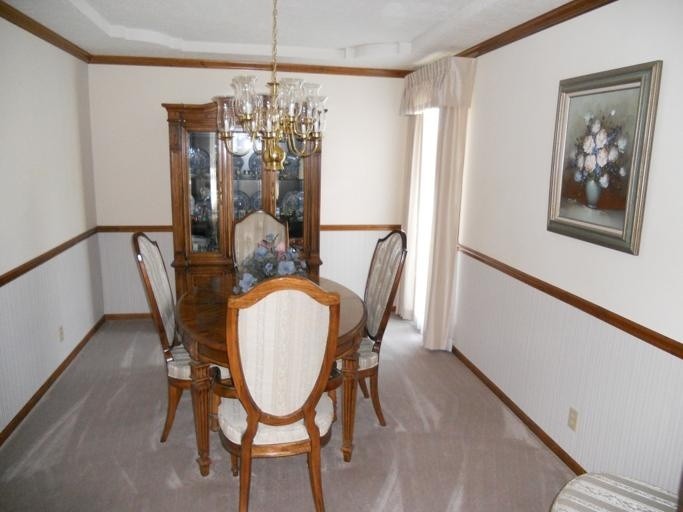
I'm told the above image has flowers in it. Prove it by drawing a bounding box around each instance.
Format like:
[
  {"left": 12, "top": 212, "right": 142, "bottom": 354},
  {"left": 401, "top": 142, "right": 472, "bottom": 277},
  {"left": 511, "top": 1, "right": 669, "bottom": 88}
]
[{"left": 574, "top": 110, "right": 629, "bottom": 188}]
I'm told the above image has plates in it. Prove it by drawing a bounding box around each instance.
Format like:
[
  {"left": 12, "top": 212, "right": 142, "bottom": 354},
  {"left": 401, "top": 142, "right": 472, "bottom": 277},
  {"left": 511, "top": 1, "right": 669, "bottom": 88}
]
[
  {"left": 247, "top": 150, "right": 264, "bottom": 173},
  {"left": 188, "top": 147, "right": 210, "bottom": 176},
  {"left": 233, "top": 190, "right": 250, "bottom": 212},
  {"left": 281, "top": 190, "right": 303, "bottom": 216},
  {"left": 250, "top": 191, "right": 262, "bottom": 212}
]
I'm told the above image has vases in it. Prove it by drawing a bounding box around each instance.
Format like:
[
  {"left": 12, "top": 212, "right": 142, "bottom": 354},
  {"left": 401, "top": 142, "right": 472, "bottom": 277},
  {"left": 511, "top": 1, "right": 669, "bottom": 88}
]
[{"left": 584, "top": 179, "right": 601, "bottom": 209}]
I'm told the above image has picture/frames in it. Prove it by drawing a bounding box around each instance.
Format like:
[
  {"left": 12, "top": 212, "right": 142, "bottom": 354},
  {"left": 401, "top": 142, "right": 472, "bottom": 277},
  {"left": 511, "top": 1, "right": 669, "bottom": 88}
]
[{"left": 546, "top": 59, "right": 664, "bottom": 256}]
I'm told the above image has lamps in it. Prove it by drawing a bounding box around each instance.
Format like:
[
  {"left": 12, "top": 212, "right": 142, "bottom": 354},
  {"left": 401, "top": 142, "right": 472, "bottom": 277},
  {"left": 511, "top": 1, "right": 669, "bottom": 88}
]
[{"left": 212, "top": 0, "right": 329, "bottom": 170}]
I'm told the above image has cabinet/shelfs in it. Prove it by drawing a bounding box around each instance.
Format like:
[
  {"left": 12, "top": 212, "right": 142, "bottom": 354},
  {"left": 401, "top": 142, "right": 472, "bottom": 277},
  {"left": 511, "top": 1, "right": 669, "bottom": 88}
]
[{"left": 161, "top": 95, "right": 323, "bottom": 303}]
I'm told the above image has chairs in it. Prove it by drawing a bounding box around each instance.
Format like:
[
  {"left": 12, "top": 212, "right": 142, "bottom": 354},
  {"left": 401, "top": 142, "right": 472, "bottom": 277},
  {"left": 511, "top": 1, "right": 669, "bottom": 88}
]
[
  {"left": 547, "top": 462, "right": 683, "bottom": 512},
  {"left": 132, "top": 230, "right": 231, "bottom": 443},
  {"left": 330, "top": 230, "right": 407, "bottom": 426},
  {"left": 207, "top": 275, "right": 341, "bottom": 512},
  {"left": 232, "top": 209, "right": 290, "bottom": 276}
]
